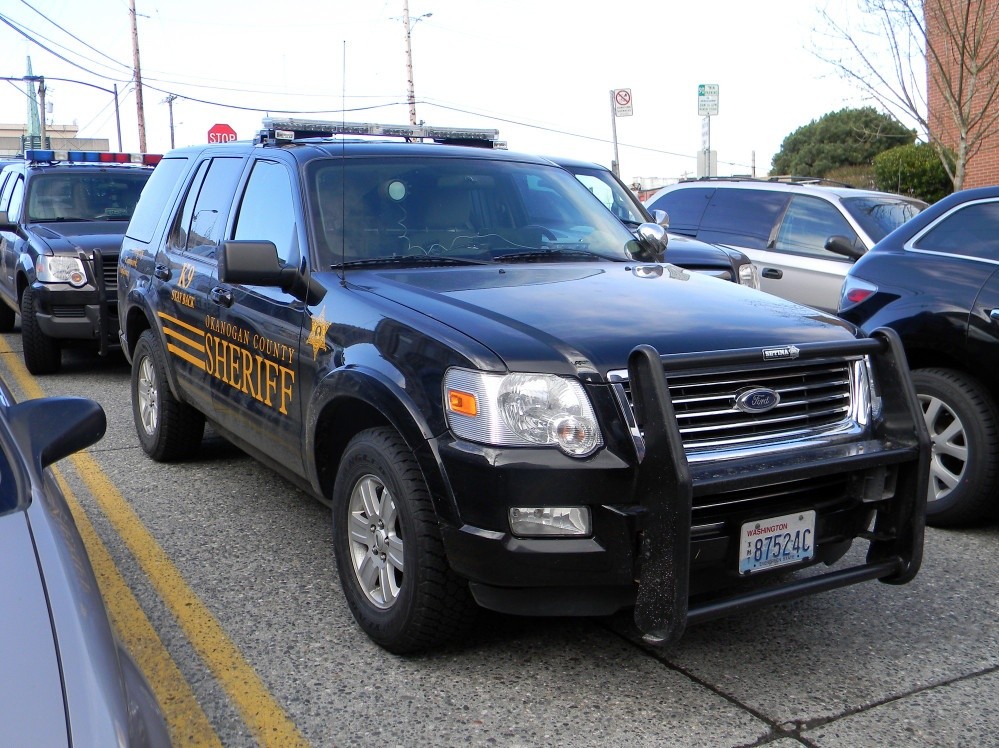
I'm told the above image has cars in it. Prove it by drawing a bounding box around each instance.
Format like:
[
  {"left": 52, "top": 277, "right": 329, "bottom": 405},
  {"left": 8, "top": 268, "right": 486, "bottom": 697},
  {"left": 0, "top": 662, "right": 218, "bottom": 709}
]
[{"left": 0, "top": 374, "right": 173, "bottom": 748}]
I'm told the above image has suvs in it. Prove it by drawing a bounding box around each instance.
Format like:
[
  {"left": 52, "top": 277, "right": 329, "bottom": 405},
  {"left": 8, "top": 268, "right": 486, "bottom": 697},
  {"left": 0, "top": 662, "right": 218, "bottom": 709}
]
[
  {"left": 641, "top": 175, "right": 933, "bottom": 319},
  {"left": 838, "top": 186, "right": 999, "bottom": 531},
  {"left": 117, "top": 116, "right": 936, "bottom": 660},
  {"left": 0, "top": 149, "right": 165, "bottom": 375},
  {"left": 547, "top": 154, "right": 760, "bottom": 291}
]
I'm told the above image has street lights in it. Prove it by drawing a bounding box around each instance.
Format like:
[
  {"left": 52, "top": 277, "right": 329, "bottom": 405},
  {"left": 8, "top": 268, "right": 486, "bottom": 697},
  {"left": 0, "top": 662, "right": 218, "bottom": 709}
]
[
  {"left": 130, "top": 12, "right": 151, "bottom": 154},
  {"left": 404, "top": 12, "right": 433, "bottom": 143}
]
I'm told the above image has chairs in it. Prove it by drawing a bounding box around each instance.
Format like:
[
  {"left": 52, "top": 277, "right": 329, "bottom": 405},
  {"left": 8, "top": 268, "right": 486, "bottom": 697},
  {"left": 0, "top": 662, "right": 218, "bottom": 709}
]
[
  {"left": 399, "top": 185, "right": 480, "bottom": 256},
  {"left": 320, "top": 190, "right": 356, "bottom": 256},
  {"left": 105, "top": 181, "right": 137, "bottom": 208},
  {"left": 32, "top": 180, "right": 67, "bottom": 218}
]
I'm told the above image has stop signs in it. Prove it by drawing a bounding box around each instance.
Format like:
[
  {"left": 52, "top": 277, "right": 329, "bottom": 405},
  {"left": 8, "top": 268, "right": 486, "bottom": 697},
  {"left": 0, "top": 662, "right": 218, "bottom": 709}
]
[{"left": 208, "top": 124, "right": 238, "bottom": 144}]
